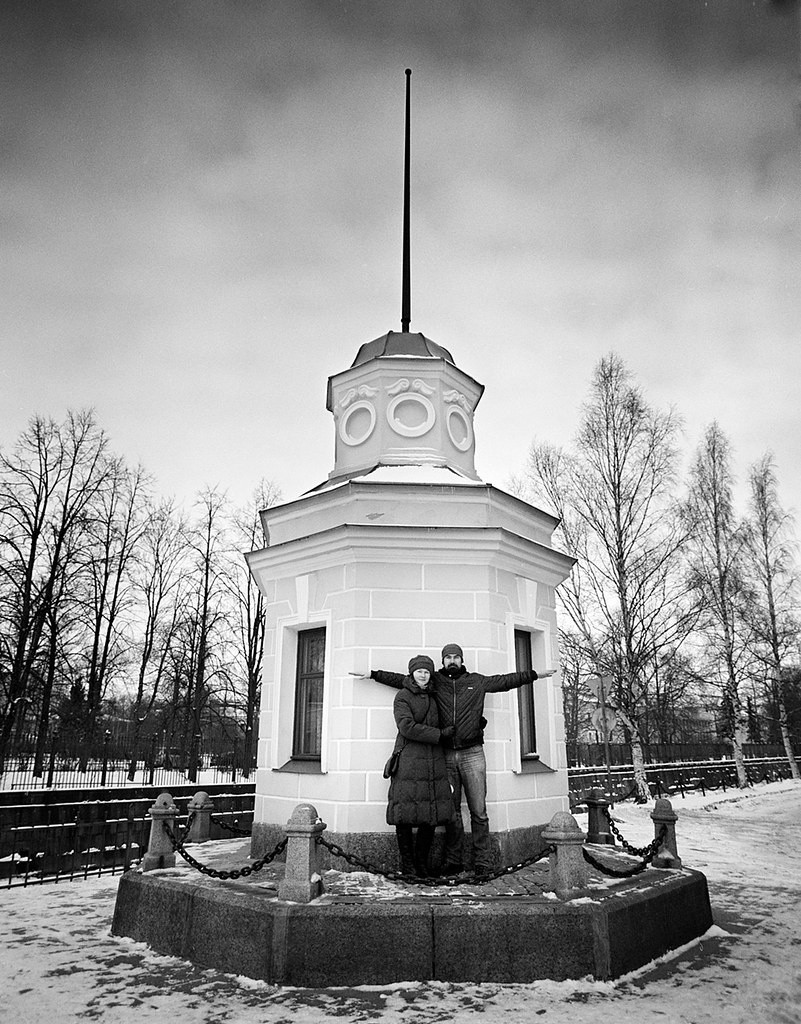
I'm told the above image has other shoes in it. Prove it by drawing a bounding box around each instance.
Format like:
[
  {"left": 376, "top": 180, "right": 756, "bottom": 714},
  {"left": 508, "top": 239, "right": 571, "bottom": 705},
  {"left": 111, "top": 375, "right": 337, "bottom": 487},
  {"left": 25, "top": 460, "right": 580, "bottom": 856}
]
[
  {"left": 447, "top": 863, "right": 464, "bottom": 875},
  {"left": 401, "top": 859, "right": 428, "bottom": 878},
  {"left": 475, "top": 865, "right": 487, "bottom": 876}
]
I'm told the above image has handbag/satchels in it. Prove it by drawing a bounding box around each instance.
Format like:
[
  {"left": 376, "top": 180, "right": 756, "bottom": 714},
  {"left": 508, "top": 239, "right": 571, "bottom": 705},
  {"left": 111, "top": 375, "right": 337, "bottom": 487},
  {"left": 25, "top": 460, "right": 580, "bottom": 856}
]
[{"left": 382, "top": 749, "right": 402, "bottom": 779}]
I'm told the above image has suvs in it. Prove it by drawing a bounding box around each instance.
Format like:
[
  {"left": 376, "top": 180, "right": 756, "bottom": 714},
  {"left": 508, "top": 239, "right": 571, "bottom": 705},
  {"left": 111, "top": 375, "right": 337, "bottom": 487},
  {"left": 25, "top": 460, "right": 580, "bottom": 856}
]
[{"left": 146, "top": 746, "right": 203, "bottom": 770}]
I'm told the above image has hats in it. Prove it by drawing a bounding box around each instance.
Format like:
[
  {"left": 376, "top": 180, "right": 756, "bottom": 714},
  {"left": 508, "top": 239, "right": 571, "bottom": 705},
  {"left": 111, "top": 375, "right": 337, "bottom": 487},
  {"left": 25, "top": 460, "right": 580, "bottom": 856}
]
[
  {"left": 408, "top": 655, "right": 434, "bottom": 677},
  {"left": 441, "top": 643, "right": 464, "bottom": 664}
]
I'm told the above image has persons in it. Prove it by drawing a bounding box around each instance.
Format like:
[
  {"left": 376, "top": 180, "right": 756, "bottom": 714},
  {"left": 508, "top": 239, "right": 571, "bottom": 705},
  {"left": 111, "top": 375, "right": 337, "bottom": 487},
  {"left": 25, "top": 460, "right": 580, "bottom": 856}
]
[{"left": 349, "top": 643, "right": 557, "bottom": 883}]
